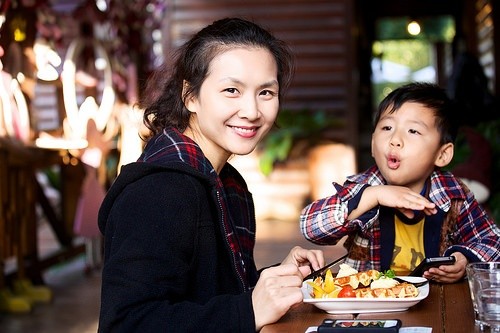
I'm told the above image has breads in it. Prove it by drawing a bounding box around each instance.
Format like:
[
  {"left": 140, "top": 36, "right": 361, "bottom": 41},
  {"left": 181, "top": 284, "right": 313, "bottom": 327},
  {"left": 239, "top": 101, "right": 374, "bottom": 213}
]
[{"left": 322, "top": 263, "right": 418, "bottom": 298}]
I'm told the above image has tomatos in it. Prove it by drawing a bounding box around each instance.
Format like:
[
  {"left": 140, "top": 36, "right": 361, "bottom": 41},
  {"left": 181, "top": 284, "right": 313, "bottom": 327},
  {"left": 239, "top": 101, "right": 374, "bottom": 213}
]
[{"left": 337, "top": 286, "right": 356, "bottom": 298}]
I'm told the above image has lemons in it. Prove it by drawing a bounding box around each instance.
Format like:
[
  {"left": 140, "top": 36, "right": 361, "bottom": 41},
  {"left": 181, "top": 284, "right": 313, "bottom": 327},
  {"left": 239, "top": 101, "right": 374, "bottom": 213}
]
[{"left": 306, "top": 269, "right": 341, "bottom": 298}]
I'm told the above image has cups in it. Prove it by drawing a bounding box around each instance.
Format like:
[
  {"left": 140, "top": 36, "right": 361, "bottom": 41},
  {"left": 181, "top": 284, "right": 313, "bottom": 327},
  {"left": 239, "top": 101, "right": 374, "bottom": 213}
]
[{"left": 465, "top": 262, "right": 500, "bottom": 333}]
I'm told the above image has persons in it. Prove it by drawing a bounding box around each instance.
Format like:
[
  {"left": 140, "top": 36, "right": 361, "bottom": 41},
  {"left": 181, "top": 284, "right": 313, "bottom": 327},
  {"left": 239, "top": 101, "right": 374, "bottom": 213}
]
[
  {"left": 97, "top": 16, "right": 324, "bottom": 333},
  {"left": 298, "top": 83, "right": 500, "bottom": 283}
]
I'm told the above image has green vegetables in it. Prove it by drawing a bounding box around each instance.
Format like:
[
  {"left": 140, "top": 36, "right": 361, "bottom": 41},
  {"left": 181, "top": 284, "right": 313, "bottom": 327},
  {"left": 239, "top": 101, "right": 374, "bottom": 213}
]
[{"left": 376, "top": 269, "right": 396, "bottom": 279}]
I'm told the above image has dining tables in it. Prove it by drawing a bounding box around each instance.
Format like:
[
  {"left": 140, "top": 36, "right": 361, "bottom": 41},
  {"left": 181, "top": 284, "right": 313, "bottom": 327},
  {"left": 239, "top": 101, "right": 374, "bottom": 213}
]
[{"left": 257, "top": 273, "right": 479, "bottom": 333}]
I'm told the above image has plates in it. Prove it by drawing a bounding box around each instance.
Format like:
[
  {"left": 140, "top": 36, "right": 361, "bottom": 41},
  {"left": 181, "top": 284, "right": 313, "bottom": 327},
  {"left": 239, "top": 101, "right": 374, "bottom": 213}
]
[{"left": 301, "top": 276, "right": 429, "bottom": 313}]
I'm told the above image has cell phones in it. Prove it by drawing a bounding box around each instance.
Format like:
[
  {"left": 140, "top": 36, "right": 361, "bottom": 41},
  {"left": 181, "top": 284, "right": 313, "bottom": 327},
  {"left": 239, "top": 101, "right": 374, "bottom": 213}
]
[
  {"left": 317, "top": 319, "right": 402, "bottom": 333},
  {"left": 410, "top": 256, "right": 455, "bottom": 276}
]
